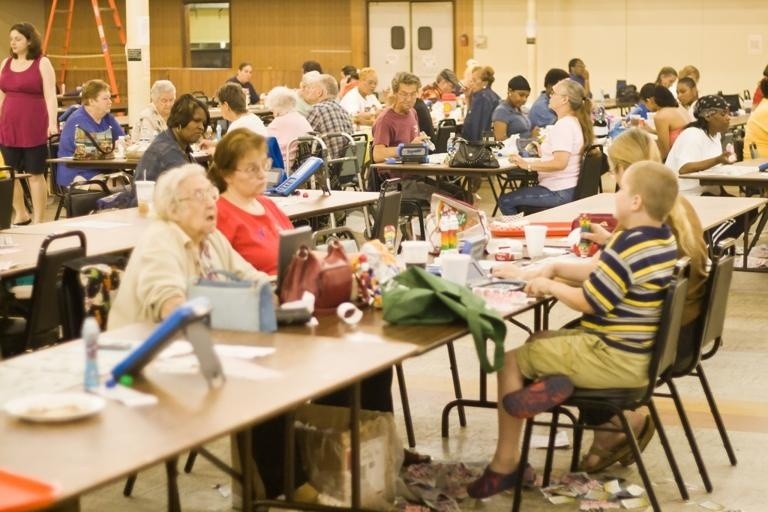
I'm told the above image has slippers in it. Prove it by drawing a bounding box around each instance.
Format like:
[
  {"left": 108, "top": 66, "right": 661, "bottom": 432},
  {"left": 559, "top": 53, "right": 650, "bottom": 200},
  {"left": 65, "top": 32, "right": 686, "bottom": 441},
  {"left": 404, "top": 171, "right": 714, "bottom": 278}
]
[
  {"left": 503, "top": 373, "right": 574, "bottom": 419},
  {"left": 465, "top": 462, "right": 533, "bottom": 499},
  {"left": 619, "top": 414, "right": 656, "bottom": 467},
  {"left": 577, "top": 440, "right": 632, "bottom": 474}
]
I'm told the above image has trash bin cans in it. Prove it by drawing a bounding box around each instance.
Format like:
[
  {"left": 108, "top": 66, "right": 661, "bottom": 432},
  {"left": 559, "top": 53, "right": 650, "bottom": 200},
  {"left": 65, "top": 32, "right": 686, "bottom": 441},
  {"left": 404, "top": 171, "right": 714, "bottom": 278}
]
[{"left": 293, "top": 404, "right": 395, "bottom": 512}]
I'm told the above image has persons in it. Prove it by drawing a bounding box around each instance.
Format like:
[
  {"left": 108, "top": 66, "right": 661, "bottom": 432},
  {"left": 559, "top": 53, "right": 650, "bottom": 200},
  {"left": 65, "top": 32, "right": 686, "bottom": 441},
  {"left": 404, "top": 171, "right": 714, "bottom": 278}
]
[{"left": 0, "top": 22, "right": 58, "bottom": 224}]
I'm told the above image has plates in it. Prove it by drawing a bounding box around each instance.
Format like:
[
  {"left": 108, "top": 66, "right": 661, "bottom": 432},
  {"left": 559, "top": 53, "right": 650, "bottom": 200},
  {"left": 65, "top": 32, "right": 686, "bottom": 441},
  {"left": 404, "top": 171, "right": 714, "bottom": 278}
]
[{"left": 6, "top": 391, "right": 102, "bottom": 423}]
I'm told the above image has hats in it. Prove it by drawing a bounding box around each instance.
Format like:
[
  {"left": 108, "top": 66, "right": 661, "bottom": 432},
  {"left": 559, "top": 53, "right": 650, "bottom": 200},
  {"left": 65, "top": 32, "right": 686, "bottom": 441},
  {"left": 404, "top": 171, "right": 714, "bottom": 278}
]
[
  {"left": 693, "top": 95, "right": 730, "bottom": 119},
  {"left": 440, "top": 68, "right": 460, "bottom": 88}
]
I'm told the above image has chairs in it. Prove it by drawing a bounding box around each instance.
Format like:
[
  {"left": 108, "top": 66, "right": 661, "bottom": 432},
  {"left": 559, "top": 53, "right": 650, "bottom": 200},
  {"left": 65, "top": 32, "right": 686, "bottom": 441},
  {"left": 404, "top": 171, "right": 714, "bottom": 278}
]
[{"left": 0, "top": 90, "right": 768, "bottom": 512}]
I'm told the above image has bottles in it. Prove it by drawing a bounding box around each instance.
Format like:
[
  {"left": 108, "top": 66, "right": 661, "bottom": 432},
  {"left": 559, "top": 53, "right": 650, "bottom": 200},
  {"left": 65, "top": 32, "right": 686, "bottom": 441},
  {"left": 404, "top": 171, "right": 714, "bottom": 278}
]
[
  {"left": 81, "top": 311, "right": 101, "bottom": 391},
  {"left": 578, "top": 217, "right": 594, "bottom": 259},
  {"left": 594, "top": 105, "right": 608, "bottom": 138},
  {"left": 425, "top": 101, "right": 433, "bottom": 117},
  {"left": 206, "top": 125, "right": 212, "bottom": 140},
  {"left": 442, "top": 92, "right": 457, "bottom": 118},
  {"left": 216, "top": 123, "right": 221, "bottom": 140},
  {"left": 438, "top": 213, "right": 458, "bottom": 250}
]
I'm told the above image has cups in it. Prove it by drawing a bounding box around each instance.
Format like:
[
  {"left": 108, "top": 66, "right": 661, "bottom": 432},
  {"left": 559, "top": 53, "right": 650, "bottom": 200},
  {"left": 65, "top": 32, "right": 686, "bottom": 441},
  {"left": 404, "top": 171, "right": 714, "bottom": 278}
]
[
  {"left": 401, "top": 240, "right": 426, "bottom": 270},
  {"left": 439, "top": 254, "right": 471, "bottom": 285},
  {"left": 523, "top": 224, "right": 548, "bottom": 259},
  {"left": 647, "top": 113, "right": 655, "bottom": 128},
  {"left": 134, "top": 180, "right": 156, "bottom": 218},
  {"left": 630, "top": 115, "right": 645, "bottom": 128}
]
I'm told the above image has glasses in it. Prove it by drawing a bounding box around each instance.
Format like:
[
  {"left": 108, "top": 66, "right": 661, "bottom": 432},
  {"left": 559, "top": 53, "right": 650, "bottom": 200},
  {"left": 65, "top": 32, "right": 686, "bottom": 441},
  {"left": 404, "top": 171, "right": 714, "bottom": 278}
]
[
  {"left": 180, "top": 186, "right": 220, "bottom": 204},
  {"left": 242, "top": 155, "right": 274, "bottom": 180}
]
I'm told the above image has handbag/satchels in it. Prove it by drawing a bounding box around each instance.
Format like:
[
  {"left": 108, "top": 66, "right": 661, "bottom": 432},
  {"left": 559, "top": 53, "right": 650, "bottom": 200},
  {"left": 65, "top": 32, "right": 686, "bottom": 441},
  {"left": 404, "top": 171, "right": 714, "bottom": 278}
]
[
  {"left": 448, "top": 139, "right": 504, "bottom": 168},
  {"left": 72, "top": 124, "right": 115, "bottom": 160},
  {"left": 185, "top": 269, "right": 278, "bottom": 333},
  {"left": 378, "top": 266, "right": 507, "bottom": 372},
  {"left": 280, "top": 236, "right": 370, "bottom": 314}
]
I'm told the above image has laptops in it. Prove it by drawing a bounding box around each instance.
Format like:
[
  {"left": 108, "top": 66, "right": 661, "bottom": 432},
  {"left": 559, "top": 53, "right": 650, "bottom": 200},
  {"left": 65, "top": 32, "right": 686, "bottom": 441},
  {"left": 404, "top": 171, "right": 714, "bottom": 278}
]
[
  {"left": 596, "top": 79, "right": 627, "bottom": 104},
  {"left": 266, "top": 225, "right": 312, "bottom": 298}
]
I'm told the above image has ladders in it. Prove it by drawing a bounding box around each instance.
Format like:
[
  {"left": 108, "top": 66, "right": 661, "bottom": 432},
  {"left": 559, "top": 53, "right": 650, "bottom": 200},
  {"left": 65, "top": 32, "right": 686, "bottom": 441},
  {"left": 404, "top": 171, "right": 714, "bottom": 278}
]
[{"left": 41, "top": 0, "right": 127, "bottom": 116}]
